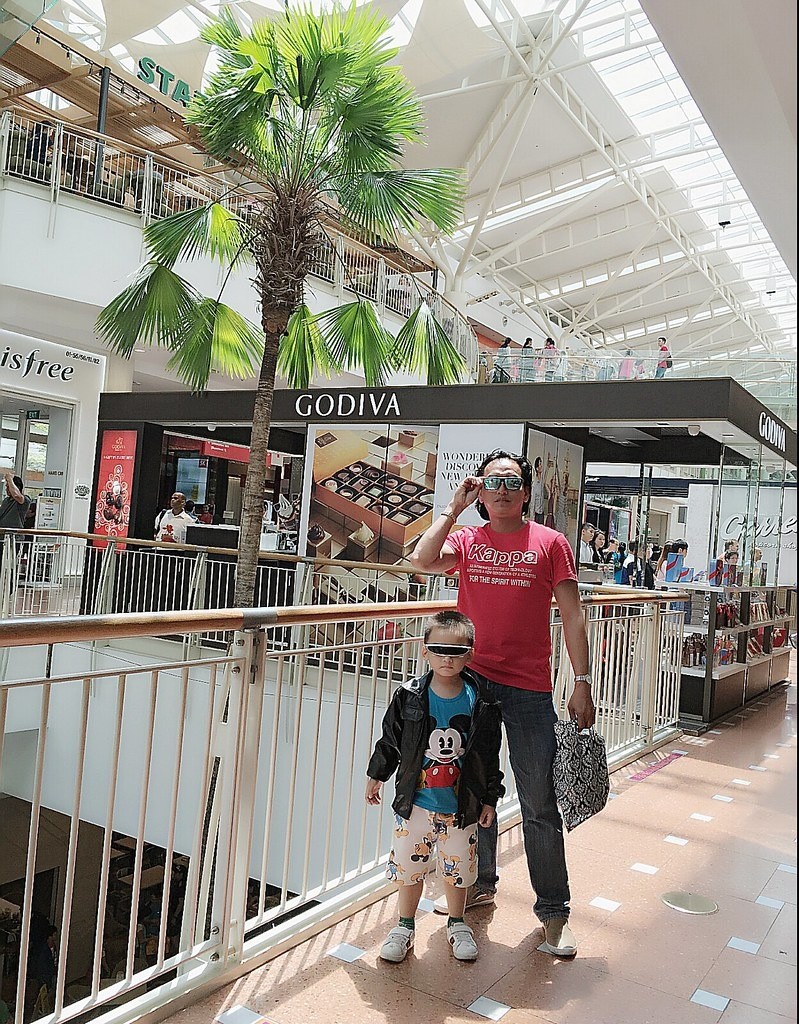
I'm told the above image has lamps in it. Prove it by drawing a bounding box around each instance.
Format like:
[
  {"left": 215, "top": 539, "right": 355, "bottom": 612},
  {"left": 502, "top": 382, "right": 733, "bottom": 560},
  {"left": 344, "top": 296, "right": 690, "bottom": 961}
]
[
  {"left": 718, "top": 182, "right": 731, "bottom": 224},
  {"left": 688, "top": 425, "right": 700, "bottom": 436},
  {"left": 765, "top": 262, "right": 776, "bottom": 295},
  {"left": 208, "top": 426, "right": 216, "bottom": 431},
  {"left": 36, "top": 32, "right": 190, "bottom": 134},
  {"left": 766, "top": 466, "right": 775, "bottom": 473}
]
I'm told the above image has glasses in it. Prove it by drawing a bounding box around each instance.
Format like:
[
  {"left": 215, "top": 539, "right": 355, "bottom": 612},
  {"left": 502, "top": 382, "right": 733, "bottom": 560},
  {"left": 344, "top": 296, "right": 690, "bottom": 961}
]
[
  {"left": 482, "top": 477, "right": 525, "bottom": 491},
  {"left": 425, "top": 643, "right": 472, "bottom": 657}
]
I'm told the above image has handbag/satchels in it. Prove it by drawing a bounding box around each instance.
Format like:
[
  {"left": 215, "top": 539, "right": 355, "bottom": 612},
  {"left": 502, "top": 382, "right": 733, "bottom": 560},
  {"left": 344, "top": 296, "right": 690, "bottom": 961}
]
[
  {"left": 666, "top": 355, "right": 672, "bottom": 368},
  {"left": 551, "top": 714, "right": 609, "bottom": 834}
]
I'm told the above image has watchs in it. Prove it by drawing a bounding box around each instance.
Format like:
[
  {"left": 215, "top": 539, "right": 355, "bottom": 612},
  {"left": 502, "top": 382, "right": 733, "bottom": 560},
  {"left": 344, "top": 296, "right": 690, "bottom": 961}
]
[{"left": 575, "top": 674, "right": 592, "bottom": 685}]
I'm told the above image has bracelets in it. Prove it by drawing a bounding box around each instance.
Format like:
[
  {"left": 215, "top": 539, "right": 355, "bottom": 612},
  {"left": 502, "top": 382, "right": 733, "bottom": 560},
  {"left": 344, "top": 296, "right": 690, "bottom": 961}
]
[
  {"left": 441, "top": 511, "right": 457, "bottom": 524},
  {"left": 174, "top": 916, "right": 177, "bottom": 918}
]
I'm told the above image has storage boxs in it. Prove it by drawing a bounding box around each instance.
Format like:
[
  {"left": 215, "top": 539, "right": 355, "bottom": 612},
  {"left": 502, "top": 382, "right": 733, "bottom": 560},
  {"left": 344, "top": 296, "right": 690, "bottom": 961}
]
[
  {"left": 312, "top": 429, "right": 435, "bottom": 546},
  {"left": 670, "top": 590, "right": 705, "bottom": 626}
]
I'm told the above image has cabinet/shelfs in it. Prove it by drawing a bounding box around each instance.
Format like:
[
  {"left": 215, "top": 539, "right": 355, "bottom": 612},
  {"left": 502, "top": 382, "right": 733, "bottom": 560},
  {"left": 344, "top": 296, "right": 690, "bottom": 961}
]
[
  {"left": 710, "top": 581, "right": 798, "bottom": 723},
  {"left": 655, "top": 581, "right": 718, "bottom": 723}
]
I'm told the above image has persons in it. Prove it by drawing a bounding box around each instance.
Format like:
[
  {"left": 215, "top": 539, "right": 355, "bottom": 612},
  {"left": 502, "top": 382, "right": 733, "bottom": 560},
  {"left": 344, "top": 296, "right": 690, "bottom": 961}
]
[
  {"left": 129, "top": 156, "right": 162, "bottom": 214},
  {"left": 579, "top": 522, "right": 660, "bottom": 683},
  {"left": 107, "top": 867, "right": 125, "bottom": 920},
  {"left": 655, "top": 539, "right": 688, "bottom": 621},
  {"left": 618, "top": 348, "right": 645, "bottom": 379},
  {"left": 128, "top": 894, "right": 160, "bottom": 959},
  {"left": 365, "top": 446, "right": 595, "bottom": 961},
  {"left": 544, "top": 466, "right": 555, "bottom": 530},
  {"left": 717, "top": 539, "right": 766, "bottom": 603},
  {"left": 91, "top": 931, "right": 111, "bottom": 979},
  {"left": 494, "top": 337, "right": 562, "bottom": 383},
  {"left": 386, "top": 274, "right": 437, "bottom": 316},
  {"left": 479, "top": 350, "right": 488, "bottom": 383},
  {"left": 654, "top": 337, "right": 668, "bottom": 377},
  {"left": 26, "top": 904, "right": 59, "bottom": 993},
  {"left": 166, "top": 865, "right": 188, "bottom": 953},
  {"left": 555, "top": 461, "right": 569, "bottom": 536},
  {"left": 238, "top": 205, "right": 252, "bottom": 234},
  {"left": 530, "top": 452, "right": 551, "bottom": 524},
  {"left": 141, "top": 853, "right": 151, "bottom": 870},
  {"left": 154, "top": 492, "right": 213, "bottom": 555},
  {"left": 0, "top": 472, "right": 32, "bottom": 602},
  {"left": 26, "top": 120, "right": 53, "bottom": 166}
]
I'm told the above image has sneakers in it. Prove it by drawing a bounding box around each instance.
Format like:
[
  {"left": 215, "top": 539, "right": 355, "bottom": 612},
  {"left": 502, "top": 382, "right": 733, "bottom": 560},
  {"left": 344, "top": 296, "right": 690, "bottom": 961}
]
[
  {"left": 543, "top": 916, "right": 578, "bottom": 955},
  {"left": 434, "top": 884, "right": 496, "bottom": 913},
  {"left": 448, "top": 921, "right": 477, "bottom": 960},
  {"left": 380, "top": 924, "right": 414, "bottom": 963}
]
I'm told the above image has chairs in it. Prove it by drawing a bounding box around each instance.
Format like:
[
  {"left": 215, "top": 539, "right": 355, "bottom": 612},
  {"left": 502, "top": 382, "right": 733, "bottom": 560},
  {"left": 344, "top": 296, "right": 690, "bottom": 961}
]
[
  {"left": 67, "top": 846, "right": 165, "bottom": 1018},
  {"left": 7, "top": 131, "right": 375, "bottom": 298}
]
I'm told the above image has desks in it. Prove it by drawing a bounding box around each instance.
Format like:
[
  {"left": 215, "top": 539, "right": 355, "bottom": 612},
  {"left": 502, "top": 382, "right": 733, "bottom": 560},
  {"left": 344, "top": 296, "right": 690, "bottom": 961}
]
[
  {"left": 119, "top": 865, "right": 164, "bottom": 889},
  {"left": 113, "top": 837, "right": 150, "bottom": 850},
  {"left": 386, "top": 289, "right": 410, "bottom": 311},
  {"left": 172, "top": 855, "right": 189, "bottom": 868},
  {"left": 63, "top": 155, "right": 96, "bottom": 194},
  {"left": 111, "top": 848, "right": 125, "bottom": 859},
  {"left": 100, "top": 979, "right": 147, "bottom": 1006}
]
